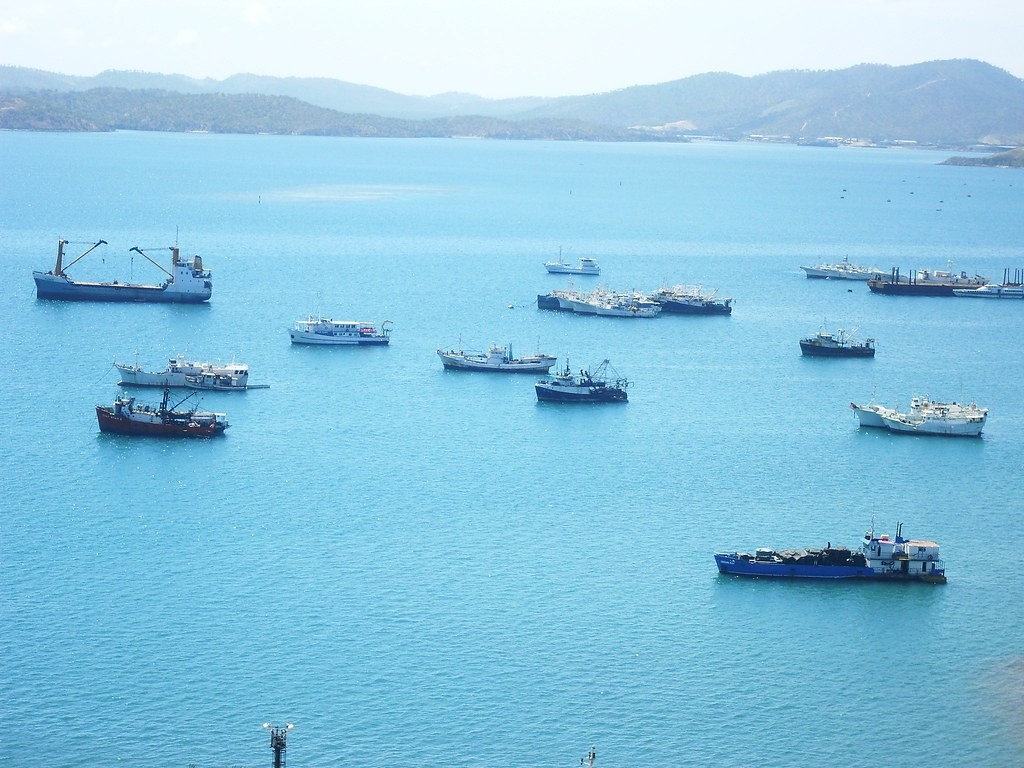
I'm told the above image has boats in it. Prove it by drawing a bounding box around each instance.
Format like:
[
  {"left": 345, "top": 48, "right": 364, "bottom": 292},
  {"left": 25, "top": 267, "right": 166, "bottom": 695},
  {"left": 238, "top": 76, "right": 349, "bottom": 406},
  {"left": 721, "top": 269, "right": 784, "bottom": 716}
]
[
  {"left": 849, "top": 393, "right": 988, "bottom": 438},
  {"left": 534, "top": 357, "right": 634, "bottom": 402},
  {"left": 32, "top": 227, "right": 215, "bottom": 303},
  {"left": 111, "top": 361, "right": 248, "bottom": 391},
  {"left": 798, "top": 317, "right": 876, "bottom": 358},
  {"left": 536, "top": 282, "right": 734, "bottom": 319},
  {"left": 94, "top": 389, "right": 230, "bottom": 439},
  {"left": 287, "top": 313, "right": 394, "bottom": 346},
  {"left": 715, "top": 521, "right": 948, "bottom": 586},
  {"left": 799, "top": 254, "right": 1024, "bottom": 299},
  {"left": 545, "top": 244, "right": 601, "bottom": 276},
  {"left": 435, "top": 333, "right": 557, "bottom": 373}
]
